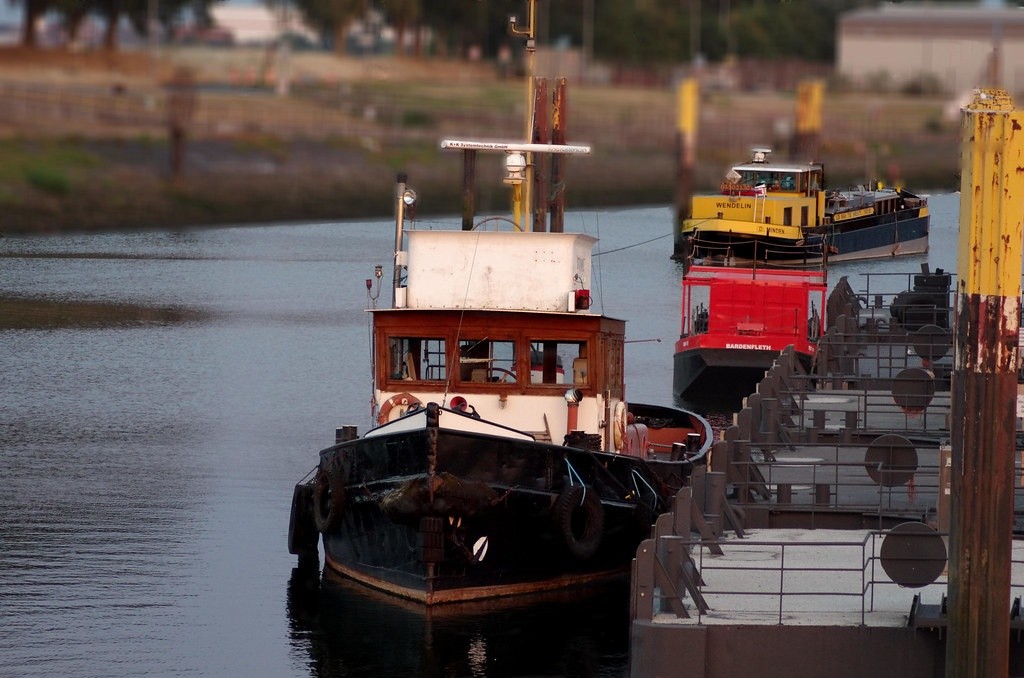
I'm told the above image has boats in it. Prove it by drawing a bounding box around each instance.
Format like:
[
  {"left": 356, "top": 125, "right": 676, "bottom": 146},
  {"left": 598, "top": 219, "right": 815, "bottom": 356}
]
[
  {"left": 673, "top": 146, "right": 932, "bottom": 403},
  {"left": 288, "top": 0, "right": 715, "bottom": 649}
]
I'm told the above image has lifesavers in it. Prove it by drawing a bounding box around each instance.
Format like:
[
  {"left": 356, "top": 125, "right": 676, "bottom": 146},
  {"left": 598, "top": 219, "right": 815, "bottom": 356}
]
[
  {"left": 639, "top": 493, "right": 665, "bottom": 533},
  {"left": 313, "top": 465, "right": 347, "bottom": 535},
  {"left": 377, "top": 392, "right": 425, "bottom": 426},
  {"left": 555, "top": 483, "right": 608, "bottom": 557},
  {"left": 614, "top": 400, "right": 628, "bottom": 450}
]
